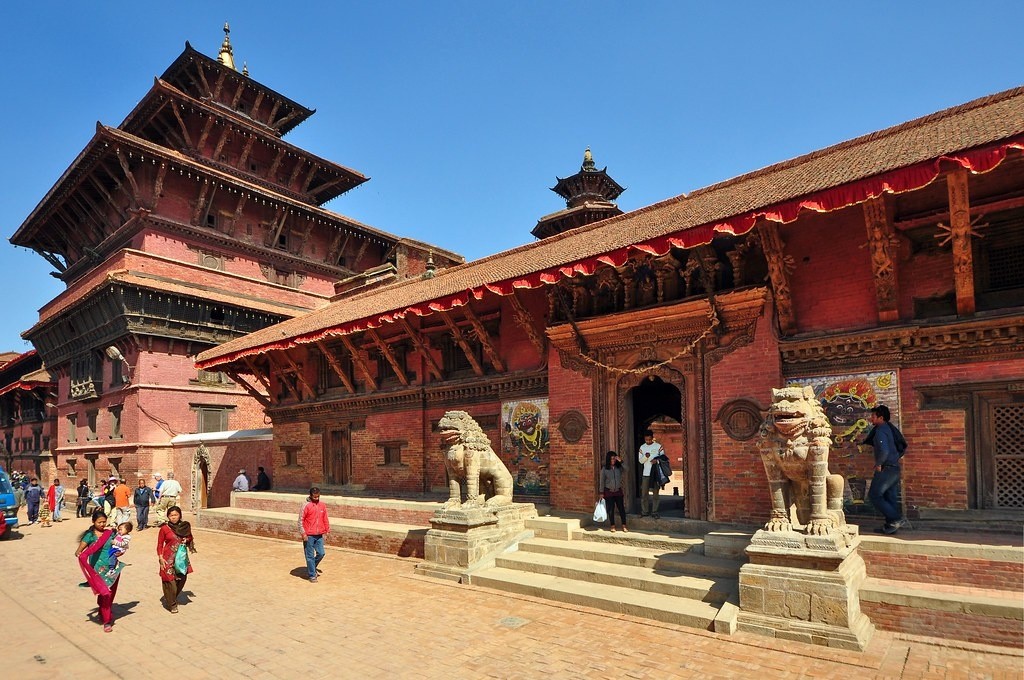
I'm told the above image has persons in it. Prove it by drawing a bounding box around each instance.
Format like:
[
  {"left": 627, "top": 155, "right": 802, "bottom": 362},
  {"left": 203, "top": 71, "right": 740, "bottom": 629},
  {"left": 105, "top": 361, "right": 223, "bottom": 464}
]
[
  {"left": 350, "top": 334, "right": 431, "bottom": 372},
  {"left": 152, "top": 473, "right": 164, "bottom": 525},
  {"left": 105, "top": 522, "right": 134, "bottom": 578},
  {"left": 452, "top": 309, "right": 536, "bottom": 351},
  {"left": 252, "top": 467, "right": 271, "bottom": 491},
  {"left": 868, "top": 405, "right": 905, "bottom": 535},
  {"left": 638, "top": 430, "right": 665, "bottom": 519},
  {"left": 134, "top": 479, "right": 155, "bottom": 530},
  {"left": 298, "top": 487, "right": 330, "bottom": 582},
  {"left": 76, "top": 478, "right": 107, "bottom": 518},
  {"left": 768, "top": 249, "right": 793, "bottom": 295},
  {"left": 23, "top": 477, "right": 65, "bottom": 527},
  {"left": 156, "top": 472, "right": 182, "bottom": 526},
  {"left": 11, "top": 471, "right": 30, "bottom": 489},
  {"left": 599, "top": 451, "right": 628, "bottom": 532},
  {"left": 12, "top": 482, "right": 25, "bottom": 528},
  {"left": 157, "top": 506, "right": 197, "bottom": 613},
  {"left": 104, "top": 474, "right": 131, "bottom": 516},
  {"left": 939, "top": 210, "right": 984, "bottom": 270},
  {"left": 252, "top": 352, "right": 341, "bottom": 384},
  {"left": 232, "top": 468, "right": 251, "bottom": 492},
  {"left": 548, "top": 232, "right": 761, "bottom": 319},
  {"left": 75, "top": 511, "right": 132, "bottom": 632},
  {"left": 870, "top": 224, "right": 893, "bottom": 277}
]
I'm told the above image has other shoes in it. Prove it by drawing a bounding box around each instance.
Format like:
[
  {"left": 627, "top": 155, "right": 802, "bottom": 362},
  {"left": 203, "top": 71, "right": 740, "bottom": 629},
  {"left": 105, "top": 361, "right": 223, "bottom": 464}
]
[
  {"left": 171, "top": 608, "right": 178, "bottom": 613},
  {"left": 653, "top": 513, "right": 660, "bottom": 519},
  {"left": 872, "top": 519, "right": 896, "bottom": 534},
  {"left": 885, "top": 517, "right": 907, "bottom": 534},
  {"left": 34, "top": 520, "right": 39, "bottom": 524},
  {"left": 610, "top": 525, "right": 616, "bottom": 531},
  {"left": 27, "top": 521, "right": 33, "bottom": 525},
  {"left": 309, "top": 577, "right": 318, "bottom": 583},
  {"left": 622, "top": 524, "right": 628, "bottom": 532},
  {"left": 104, "top": 623, "right": 112, "bottom": 632},
  {"left": 638, "top": 513, "right": 645, "bottom": 518}
]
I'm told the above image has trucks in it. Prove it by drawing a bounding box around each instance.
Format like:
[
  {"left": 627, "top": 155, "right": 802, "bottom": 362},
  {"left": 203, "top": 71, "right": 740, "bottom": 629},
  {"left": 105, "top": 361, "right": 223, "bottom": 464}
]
[{"left": 0, "top": 466, "right": 19, "bottom": 540}]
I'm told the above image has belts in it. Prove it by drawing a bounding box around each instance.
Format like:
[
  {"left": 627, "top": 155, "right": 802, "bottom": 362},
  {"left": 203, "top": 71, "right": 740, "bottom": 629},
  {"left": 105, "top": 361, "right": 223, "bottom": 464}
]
[{"left": 161, "top": 495, "right": 175, "bottom": 497}]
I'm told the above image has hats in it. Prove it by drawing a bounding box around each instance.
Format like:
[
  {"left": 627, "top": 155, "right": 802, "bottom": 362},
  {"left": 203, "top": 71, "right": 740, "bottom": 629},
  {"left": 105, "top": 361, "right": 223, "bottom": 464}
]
[
  {"left": 154, "top": 473, "right": 160, "bottom": 477},
  {"left": 109, "top": 477, "right": 118, "bottom": 484},
  {"left": 238, "top": 469, "right": 246, "bottom": 473}
]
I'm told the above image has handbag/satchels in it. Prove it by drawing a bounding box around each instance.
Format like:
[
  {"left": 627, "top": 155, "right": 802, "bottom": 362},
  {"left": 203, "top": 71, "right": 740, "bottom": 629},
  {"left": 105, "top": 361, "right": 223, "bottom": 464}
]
[
  {"left": 593, "top": 498, "right": 607, "bottom": 522},
  {"left": 77, "top": 497, "right": 83, "bottom": 505},
  {"left": 174, "top": 542, "right": 188, "bottom": 576}
]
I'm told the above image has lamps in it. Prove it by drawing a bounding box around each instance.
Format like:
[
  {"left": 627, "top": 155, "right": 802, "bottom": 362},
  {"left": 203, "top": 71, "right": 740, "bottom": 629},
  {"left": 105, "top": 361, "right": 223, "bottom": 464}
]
[
  {"left": 107, "top": 346, "right": 135, "bottom": 384},
  {"left": 45, "top": 403, "right": 58, "bottom": 409}
]
